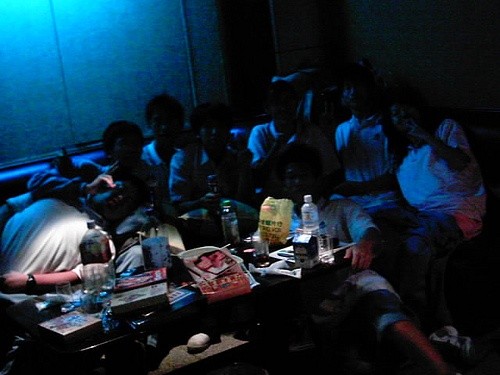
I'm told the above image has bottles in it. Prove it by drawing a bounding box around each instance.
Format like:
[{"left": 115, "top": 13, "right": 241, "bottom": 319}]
[
  {"left": 79, "top": 220, "right": 115, "bottom": 298},
  {"left": 221, "top": 200, "right": 241, "bottom": 250},
  {"left": 301, "top": 194, "right": 320, "bottom": 239}
]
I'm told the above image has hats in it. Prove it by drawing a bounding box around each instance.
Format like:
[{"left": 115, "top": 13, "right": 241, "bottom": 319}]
[{"left": 273, "top": 138, "right": 327, "bottom": 176}]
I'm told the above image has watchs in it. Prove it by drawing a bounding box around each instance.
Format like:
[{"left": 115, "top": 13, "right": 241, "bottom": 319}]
[{"left": 26, "top": 273, "right": 37, "bottom": 294}]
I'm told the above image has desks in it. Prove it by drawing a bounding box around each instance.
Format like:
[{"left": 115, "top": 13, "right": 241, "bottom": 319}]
[{"left": 25, "top": 235, "right": 380, "bottom": 375}]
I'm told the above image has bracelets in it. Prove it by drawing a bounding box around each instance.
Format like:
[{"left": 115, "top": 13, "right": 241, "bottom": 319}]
[{"left": 431, "top": 140, "right": 442, "bottom": 155}]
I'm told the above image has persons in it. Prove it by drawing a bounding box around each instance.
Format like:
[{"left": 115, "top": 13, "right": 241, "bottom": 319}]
[{"left": 0, "top": 69, "right": 486, "bottom": 375}]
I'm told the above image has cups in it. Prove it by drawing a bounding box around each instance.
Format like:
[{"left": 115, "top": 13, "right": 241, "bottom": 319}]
[
  {"left": 79, "top": 266, "right": 96, "bottom": 294},
  {"left": 318, "top": 235, "right": 334, "bottom": 259},
  {"left": 56, "top": 281, "right": 74, "bottom": 301},
  {"left": 250, "top": 230, "right": 270, "bottom": 268}
]
